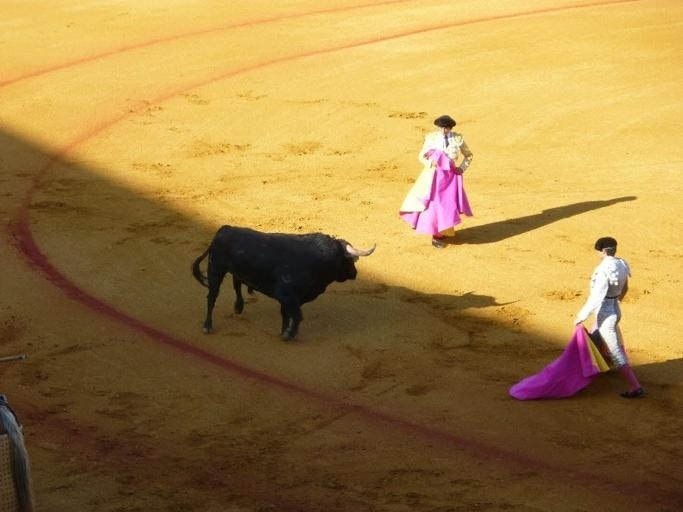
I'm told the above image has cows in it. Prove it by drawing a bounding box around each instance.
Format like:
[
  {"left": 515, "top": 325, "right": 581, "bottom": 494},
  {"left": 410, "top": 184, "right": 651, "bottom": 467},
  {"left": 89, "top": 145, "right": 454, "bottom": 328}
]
[{"left": 189, "top": 225, "right": 377, "bottom": 341}]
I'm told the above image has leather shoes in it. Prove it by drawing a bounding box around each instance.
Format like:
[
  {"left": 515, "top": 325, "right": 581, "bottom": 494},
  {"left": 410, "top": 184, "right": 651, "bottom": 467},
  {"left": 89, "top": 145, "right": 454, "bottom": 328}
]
[
  {"left": 431, "top": 236, "right": 447, "bottom": 249},
  {"left": 619, "top": 387, "right": 644, "bottom": 399}
]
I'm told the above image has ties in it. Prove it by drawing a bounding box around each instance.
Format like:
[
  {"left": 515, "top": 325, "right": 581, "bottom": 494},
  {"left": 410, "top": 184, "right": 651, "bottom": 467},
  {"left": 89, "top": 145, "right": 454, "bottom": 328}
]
[{"left": 444, "top": 134, "right": 449, "bottom": 148}]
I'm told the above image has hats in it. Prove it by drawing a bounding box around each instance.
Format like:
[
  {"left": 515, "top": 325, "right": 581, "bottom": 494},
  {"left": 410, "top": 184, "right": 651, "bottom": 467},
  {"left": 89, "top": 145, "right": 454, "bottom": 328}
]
[
  {"left": 594, "top": 236, "right": 618, "bottom": 252},
  {"left": 433, "top": 114, "right": 457, "bottom": 129}
]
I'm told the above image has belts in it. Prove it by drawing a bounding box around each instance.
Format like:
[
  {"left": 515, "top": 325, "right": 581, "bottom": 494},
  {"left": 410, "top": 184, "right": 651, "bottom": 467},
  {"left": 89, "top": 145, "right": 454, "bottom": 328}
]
[{"left": 606, "top": 294, "right": 619, "bottom": 300}]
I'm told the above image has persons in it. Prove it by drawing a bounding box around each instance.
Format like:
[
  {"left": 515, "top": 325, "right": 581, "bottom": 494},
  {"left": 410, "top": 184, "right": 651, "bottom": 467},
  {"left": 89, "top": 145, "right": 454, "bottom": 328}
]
[
  {"left": 419, "top": 115, "right": 473, "bottom": 248},
  {"left": 575, "top": 236, "right": 644, "bottom": 398}
]
